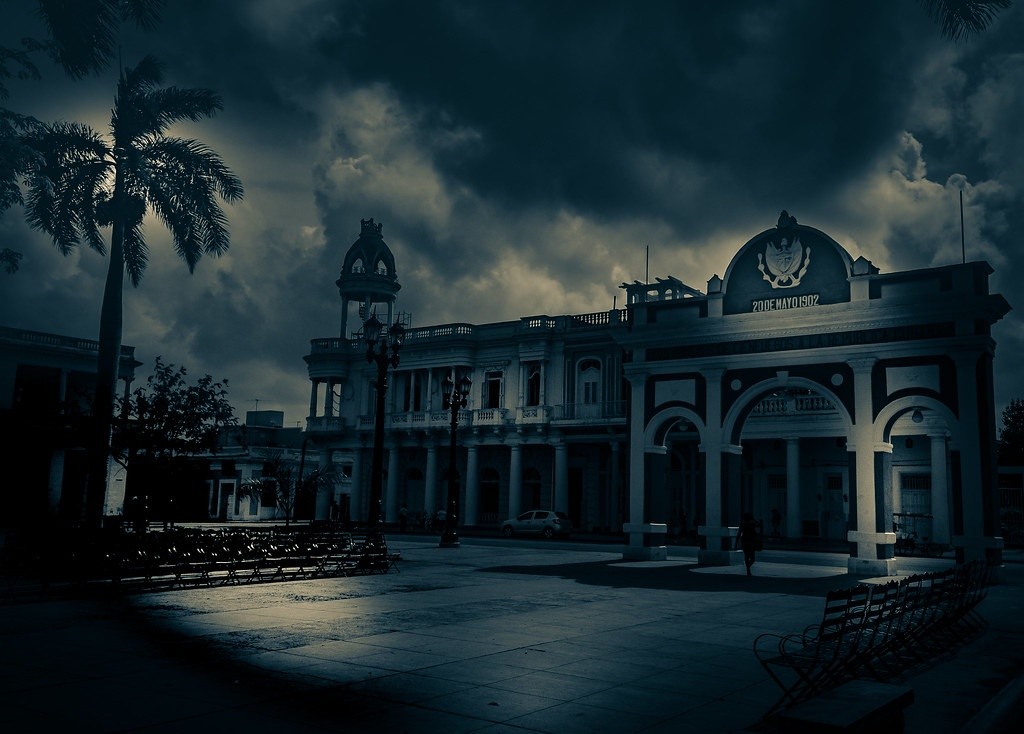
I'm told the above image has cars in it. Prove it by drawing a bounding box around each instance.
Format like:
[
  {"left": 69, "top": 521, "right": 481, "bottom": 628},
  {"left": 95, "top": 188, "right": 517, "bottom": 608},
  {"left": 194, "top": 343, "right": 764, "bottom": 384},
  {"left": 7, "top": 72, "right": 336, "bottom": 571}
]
[{"left": 501, "top": 510, "right": 572, "bottom": 541}]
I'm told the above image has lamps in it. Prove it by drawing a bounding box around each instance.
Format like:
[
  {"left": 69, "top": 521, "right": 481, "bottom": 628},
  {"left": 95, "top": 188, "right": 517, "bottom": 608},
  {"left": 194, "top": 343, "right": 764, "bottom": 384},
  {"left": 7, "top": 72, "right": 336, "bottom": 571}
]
[{"left": 912, "top": 410, "right": 923, "bottom": 423}]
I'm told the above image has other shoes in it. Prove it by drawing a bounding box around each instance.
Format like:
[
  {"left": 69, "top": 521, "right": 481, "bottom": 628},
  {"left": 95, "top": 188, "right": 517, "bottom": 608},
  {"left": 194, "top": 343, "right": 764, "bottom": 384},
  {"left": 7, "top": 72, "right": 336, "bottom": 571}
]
[{"left": 747, "top": 572, "right": 751, "bottom": 576}]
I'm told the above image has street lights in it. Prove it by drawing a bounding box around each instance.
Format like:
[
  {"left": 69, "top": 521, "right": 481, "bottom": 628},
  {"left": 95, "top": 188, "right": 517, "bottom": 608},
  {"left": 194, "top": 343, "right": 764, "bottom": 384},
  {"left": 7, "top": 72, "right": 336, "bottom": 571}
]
[
  {"left": 438, "top": 373, "right": 474, "bottom": 548},
  {"left": 362, "top": 313, "right": 405, "bottom": 555}
]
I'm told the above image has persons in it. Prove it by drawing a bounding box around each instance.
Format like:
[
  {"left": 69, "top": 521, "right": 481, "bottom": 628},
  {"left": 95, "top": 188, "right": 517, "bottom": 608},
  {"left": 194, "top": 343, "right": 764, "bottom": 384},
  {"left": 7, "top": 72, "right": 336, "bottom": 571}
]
[
  {"left": 331, "top": 500, "right": 340, "bottom": 522},
  {"left": 399, "top": 503, "right": 409, "bottom": 533},
  {"left": 679, "top": 509, "right": 688, "bottom": 537},
  {"left": 733, "top": 513, "right": 763, "bottom": 576},
  {"left": 438, "top": 507, "right": 447, "bottom": 532}
]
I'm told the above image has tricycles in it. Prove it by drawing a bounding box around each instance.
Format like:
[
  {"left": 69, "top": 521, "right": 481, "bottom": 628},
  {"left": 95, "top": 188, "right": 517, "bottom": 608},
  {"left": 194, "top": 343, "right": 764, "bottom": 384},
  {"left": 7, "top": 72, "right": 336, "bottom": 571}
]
[{"left": 893, "top": 512, "right": 934, "bottom": 556}]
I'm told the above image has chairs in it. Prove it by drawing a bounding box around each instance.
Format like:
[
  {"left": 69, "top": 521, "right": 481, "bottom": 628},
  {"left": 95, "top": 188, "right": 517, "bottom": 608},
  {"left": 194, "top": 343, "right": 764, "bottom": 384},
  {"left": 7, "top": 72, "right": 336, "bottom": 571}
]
[
  {"left": 744, "top": 558, "right": 995, "bottom": 729},
  {"left": 138, "top": 524, "right": 404, "bottom": 591}
]
